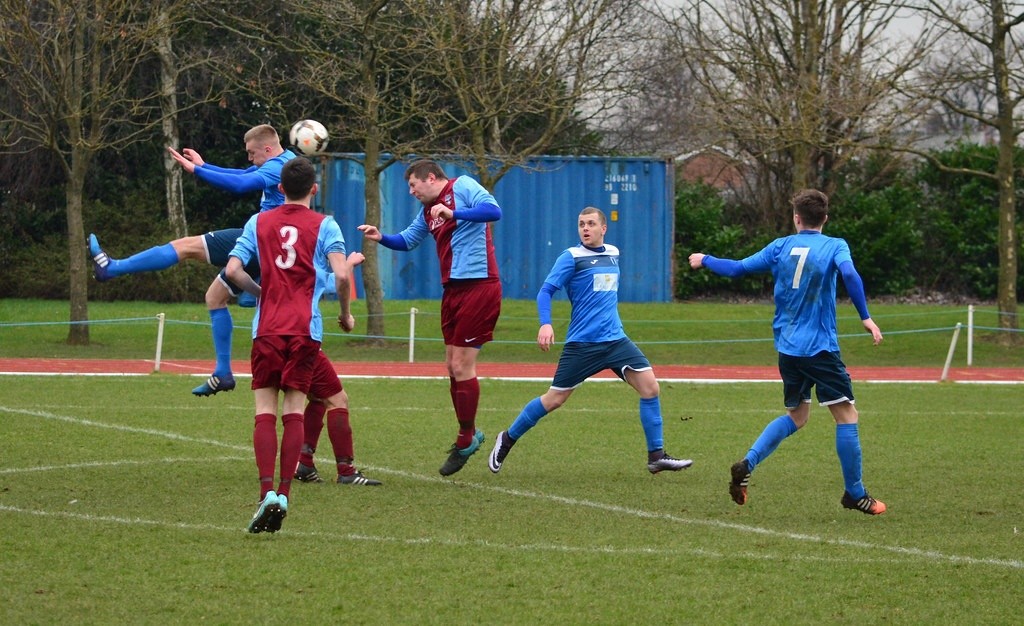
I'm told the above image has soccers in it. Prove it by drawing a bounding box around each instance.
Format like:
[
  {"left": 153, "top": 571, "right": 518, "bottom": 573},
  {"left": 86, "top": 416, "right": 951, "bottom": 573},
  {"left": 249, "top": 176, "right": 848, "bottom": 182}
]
[{"left": 289, "top": 119, "right": 329, "bottom": 157}]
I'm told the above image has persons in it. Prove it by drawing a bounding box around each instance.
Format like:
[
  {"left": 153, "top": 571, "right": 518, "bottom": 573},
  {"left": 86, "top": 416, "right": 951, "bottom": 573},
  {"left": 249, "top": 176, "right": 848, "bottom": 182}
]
[
  {"left": 224, "top": 155, "right": 355, "bottom": 535},
  {"left": 490, "top": 206, "right": 694, "bottom": 473},
  {"left": 292, "top": 252, "right": 383, "bottom": 485},
  {"left": 92, "top": 123, "right": 296, "bottom": 396},
  {"left": 358, "top": 160, "right": 503, "bottom": 476},
  {"left": 689, "top": 189, "right": 886, "bottom": 514}
]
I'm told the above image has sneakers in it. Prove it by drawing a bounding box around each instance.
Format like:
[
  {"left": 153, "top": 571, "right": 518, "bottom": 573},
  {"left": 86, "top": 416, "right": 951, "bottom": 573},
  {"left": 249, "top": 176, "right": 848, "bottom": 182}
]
[
  {"left": 248, "top": 491, "right": 280, "bottom": 533},
  {"left": 488, "top": 431, "right": 510, "bottom": 473},
  {"left": 729, "top": 460, "right": 751, "bottom": 505},
  {"left": 841, "top": 489, "right": 886, "bottom": 515},
  {"left": 475, "top": 428, "right": 486, "bottom": 444},
  {"left": 648, "top": 450, "right": 693, "bottom": 473},
  {"left": 337, "top": 469, "right": 383, "bottom": 485},
  {"left": 293, "top": 461, "right": 324, "bottom": 483},
  {"left": 266, "top": 494, "right": 287, "bottom": 534},
  {"left": 86, "top": 234, "right": 111, "bottom": 282},
  {"left": 440, "top": 436, "right": 480, "bottom": 476},
  {"left": 192, "top": 372, "right": 235, "bottom": 397}
]
